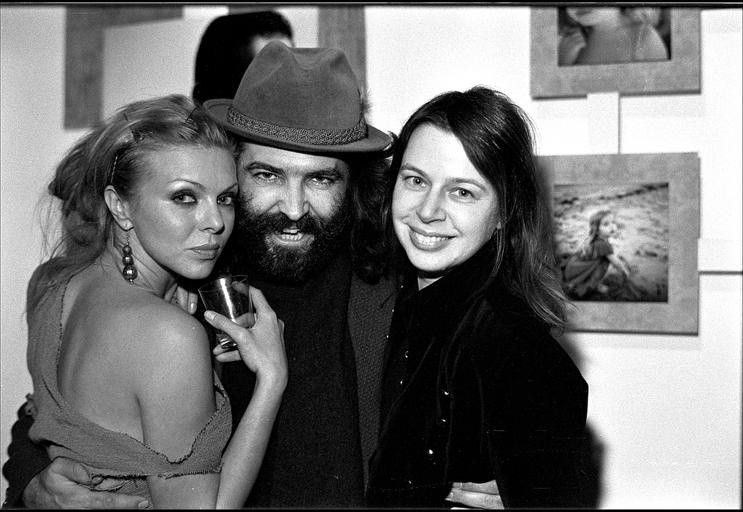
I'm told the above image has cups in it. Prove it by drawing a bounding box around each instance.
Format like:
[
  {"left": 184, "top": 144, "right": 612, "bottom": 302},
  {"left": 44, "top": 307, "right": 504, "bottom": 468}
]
[{"left": 198, "top": 275, "right": 255, "bottom": 350}]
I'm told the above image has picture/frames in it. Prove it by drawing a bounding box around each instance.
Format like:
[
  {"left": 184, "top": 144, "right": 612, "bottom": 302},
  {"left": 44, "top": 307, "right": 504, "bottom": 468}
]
[
  {"left": 522, "top": 3, "right": 706, "bottom": 102},
  {"left": 531, "top": 147, "right": 704, "bottom": 341}
]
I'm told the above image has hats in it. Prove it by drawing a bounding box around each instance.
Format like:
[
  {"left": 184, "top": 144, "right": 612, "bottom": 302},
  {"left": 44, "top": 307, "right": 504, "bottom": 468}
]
[{"left": 203, "top": 41, "right": 391, "bottom": 153}]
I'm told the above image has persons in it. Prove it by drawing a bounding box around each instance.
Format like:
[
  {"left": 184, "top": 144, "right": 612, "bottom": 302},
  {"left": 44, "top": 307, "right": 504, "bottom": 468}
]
[
  {"left": 0, "top": 39, "right": 511, "bottom": 512},
  {"left": 555, "top": 4, "right": 669, "bottom": 69},
  {"left": 561, "top": 205, "right": 631, "bottom": 300},
  {"left": 20, "top": 88, "right": 290, "bottom": 510},
  {"left": 358, "top": 81, "right": 600, "bottom": 509},
  {"left": 188, "top": 8, "right": 301, "bottom": 115}
]
